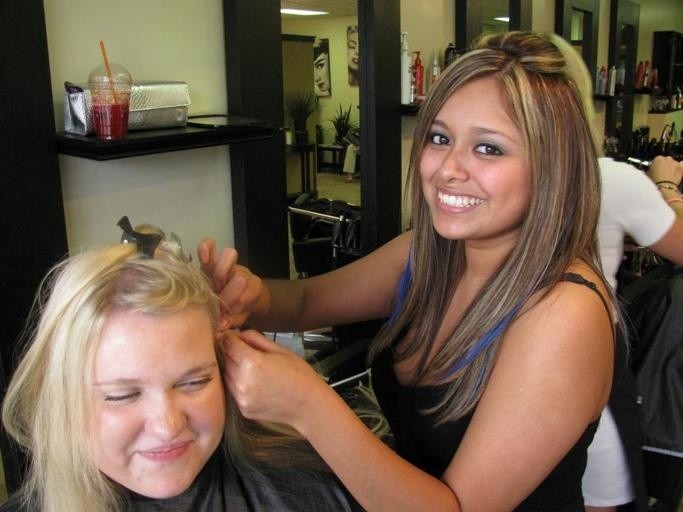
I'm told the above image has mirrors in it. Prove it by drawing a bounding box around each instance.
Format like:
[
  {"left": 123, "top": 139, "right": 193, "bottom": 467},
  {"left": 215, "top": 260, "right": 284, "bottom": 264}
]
[
  {"left": 556, "top": 0, "right": 601, "bottom": 100},
  {"left": 455, "top": 0, "right": 532, "bottom": 57},
  {"left": 606, "top": 0, "right": 641, "bottom": 159},
  {"left": 223, "top": 1, "right": 402, "bottom": 391}
]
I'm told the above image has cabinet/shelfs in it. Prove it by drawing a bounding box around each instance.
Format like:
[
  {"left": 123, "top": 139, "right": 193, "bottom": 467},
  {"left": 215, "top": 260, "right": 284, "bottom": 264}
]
[{"left": 648, "top": 31, "right": 682, "bottom": 114}]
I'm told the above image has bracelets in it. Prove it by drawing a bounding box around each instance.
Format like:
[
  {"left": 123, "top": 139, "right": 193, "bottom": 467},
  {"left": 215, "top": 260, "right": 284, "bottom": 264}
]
[
  {"left": 654, "top": 179, "right": 679, "bottom": 188},
  {"left": 663, "top": 191, "right": 683, "bottom": 208},
  {"left": 657, "top": 183, "right": 682, "bottom": 195}
]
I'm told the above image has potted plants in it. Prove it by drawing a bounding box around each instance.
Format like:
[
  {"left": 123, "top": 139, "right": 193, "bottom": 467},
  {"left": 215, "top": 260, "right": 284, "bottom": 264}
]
[
  {"left": 328, "top": 102, "right": 353, "bottom": 145},
  {"left": 283, "top": 92, "right": 320, "bottom": 146}
]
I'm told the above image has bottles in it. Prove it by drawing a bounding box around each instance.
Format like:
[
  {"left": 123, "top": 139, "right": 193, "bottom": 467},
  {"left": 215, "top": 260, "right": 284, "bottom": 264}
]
[
  {"left": 596, "top": 61, "right": 651, "bottom": 98},
  {"left": 444, "top": 44, "right": 456, "bottom": 70}
]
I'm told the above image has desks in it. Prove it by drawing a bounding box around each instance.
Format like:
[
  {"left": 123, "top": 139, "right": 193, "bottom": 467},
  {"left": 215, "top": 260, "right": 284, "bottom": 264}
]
[
  {"left": 320, "top": 144, "right": 346, "bottom": 175},
  {"left": 287, "top": 145, "right": 319, "bottom": 212}
]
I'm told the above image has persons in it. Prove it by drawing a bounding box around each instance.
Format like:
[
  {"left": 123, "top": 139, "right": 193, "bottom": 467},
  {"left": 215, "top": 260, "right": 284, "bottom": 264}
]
[
  {"left": 0, "top": 224, "right": 357, "bottom": 511},
  {"left": 195, "top": 29, "right": 618, "bottom": 512},
  {"left": 311, "top": 38, "right": 330, "bottom": 93},
  {"left": 533, "top": 33, "right": 683, "bottom": 511},
  {"left": 346, "top": 28, "right": 359, "bottom": 85}
]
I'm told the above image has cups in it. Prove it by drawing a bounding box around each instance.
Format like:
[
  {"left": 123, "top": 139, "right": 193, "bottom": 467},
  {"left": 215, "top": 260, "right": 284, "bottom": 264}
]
[{"left": 87, "top": 63, "right": 132, "bottom": 143}]
